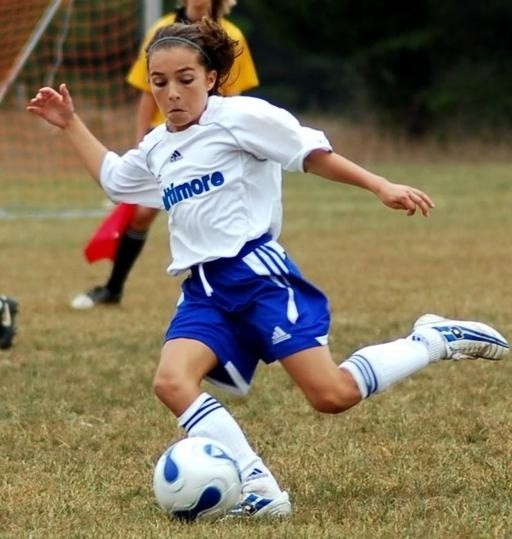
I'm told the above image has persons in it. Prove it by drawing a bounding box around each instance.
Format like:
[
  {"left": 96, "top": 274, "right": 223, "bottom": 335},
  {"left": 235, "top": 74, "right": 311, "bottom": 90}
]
[
  {"left": 71, "top": 1, "right": 260, "bottom": 314},
  {"left": 26, "top": 16, "right": 510, "bottom": 520}
]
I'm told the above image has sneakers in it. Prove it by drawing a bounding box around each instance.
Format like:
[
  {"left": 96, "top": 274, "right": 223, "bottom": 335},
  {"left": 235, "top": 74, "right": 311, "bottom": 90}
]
[
  {"left": 0, "top": 297, "right": 17, "bottom": 350},
  {"left": 69, "top": 288, "right": 120, "bottom": 311},
  {"left": 413, "top": 314, "right": 508, "bottom": 361},
  {"left": 218, "top": 490, "right": 292, "bottom": 523}
]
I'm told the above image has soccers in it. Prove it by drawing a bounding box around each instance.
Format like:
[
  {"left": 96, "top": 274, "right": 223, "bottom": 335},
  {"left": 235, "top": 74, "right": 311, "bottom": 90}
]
[{"left": 152, "top": 434, "right": 242, "bottom": 524}]
